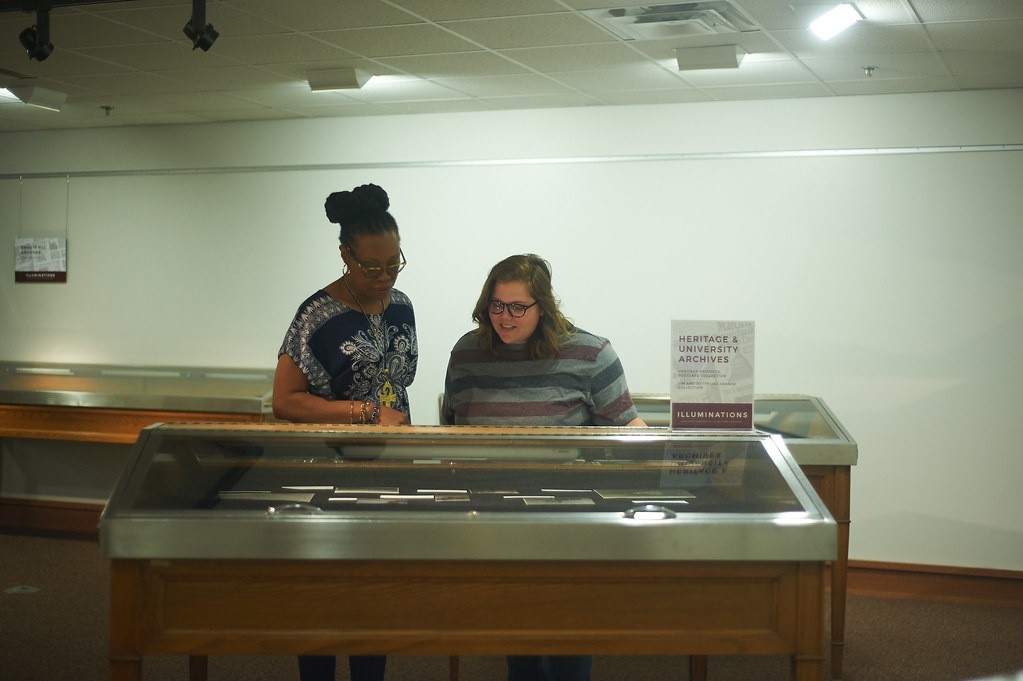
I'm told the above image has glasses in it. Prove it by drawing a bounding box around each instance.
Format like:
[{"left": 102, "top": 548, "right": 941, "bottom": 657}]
[
  {"left": 345, "top": 245, "right": 408, "bottom": 278},
  {"left": 487, "top": 298, "right": 537, "bottom": 317}
]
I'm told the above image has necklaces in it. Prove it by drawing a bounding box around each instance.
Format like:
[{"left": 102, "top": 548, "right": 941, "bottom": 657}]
[{"left": 343, "top": 274, "right": 391, "bottom": 377}]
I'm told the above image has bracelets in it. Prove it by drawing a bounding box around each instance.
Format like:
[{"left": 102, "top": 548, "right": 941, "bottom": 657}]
[
  {"left": 360, "top": 398, "right": 369, "bottom": 424},
  {"left": 349, "top": 399, "right": 354, "bottom": 424},
  {"left": 370, "top": 399, "right": 380, "bottom": 425}
]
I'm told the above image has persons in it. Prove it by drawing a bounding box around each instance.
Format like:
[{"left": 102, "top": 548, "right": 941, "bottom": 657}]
[
  {"left": 442, "top": 254, "right": 646, "bottom": 428},
  {"left": 271, "top": 183, "right": 418, "bottom": 430}
]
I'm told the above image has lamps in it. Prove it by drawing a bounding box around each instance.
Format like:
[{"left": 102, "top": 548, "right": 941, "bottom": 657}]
[
  {"left": 182, "top": 0, "right": 219, "bottom": 53},
  {"left": 18, "top": 4, "right": 54, "bottom": 62},
  {"left": 0, "top": 81, "right": 33, "bottom": 104},
  {"left": 791, "top": 4, "right": 863, "bottom": 40},
  {"left": 305, "top": 69, "right": 374, "bottom": 91},
  {"left": 672, "top": 43, "right": 745, "bottom": 71}
]
